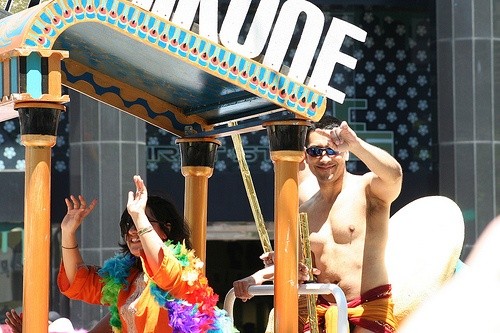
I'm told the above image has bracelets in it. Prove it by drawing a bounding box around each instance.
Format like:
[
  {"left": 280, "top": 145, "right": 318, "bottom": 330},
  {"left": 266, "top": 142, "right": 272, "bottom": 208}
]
[
  {"left": 138, "top": 225, "right": 153, "bottom": 235},
  {"left": 61, "top": 244, "right": 78, "bottom": 249}
]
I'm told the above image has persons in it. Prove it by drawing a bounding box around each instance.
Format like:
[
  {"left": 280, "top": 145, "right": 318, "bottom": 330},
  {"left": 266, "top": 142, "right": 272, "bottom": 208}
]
[
  {"left": 298, "top": 115, "right": 402, "bottom": 333},
  {"left": 57, "top": 175, "right": 239, "bottom": 333},
  {"left": 234, "top": 147, "right": 319, "bottom": 301}
]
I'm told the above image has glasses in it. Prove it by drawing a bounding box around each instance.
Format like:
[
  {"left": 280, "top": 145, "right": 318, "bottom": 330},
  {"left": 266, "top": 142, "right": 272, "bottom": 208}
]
[
  {"left": 124, "top": 219, "right": 162, "bottom": 231},
  {"left": 305, "top": 147, "right": 338, "bottom": 157}
]
